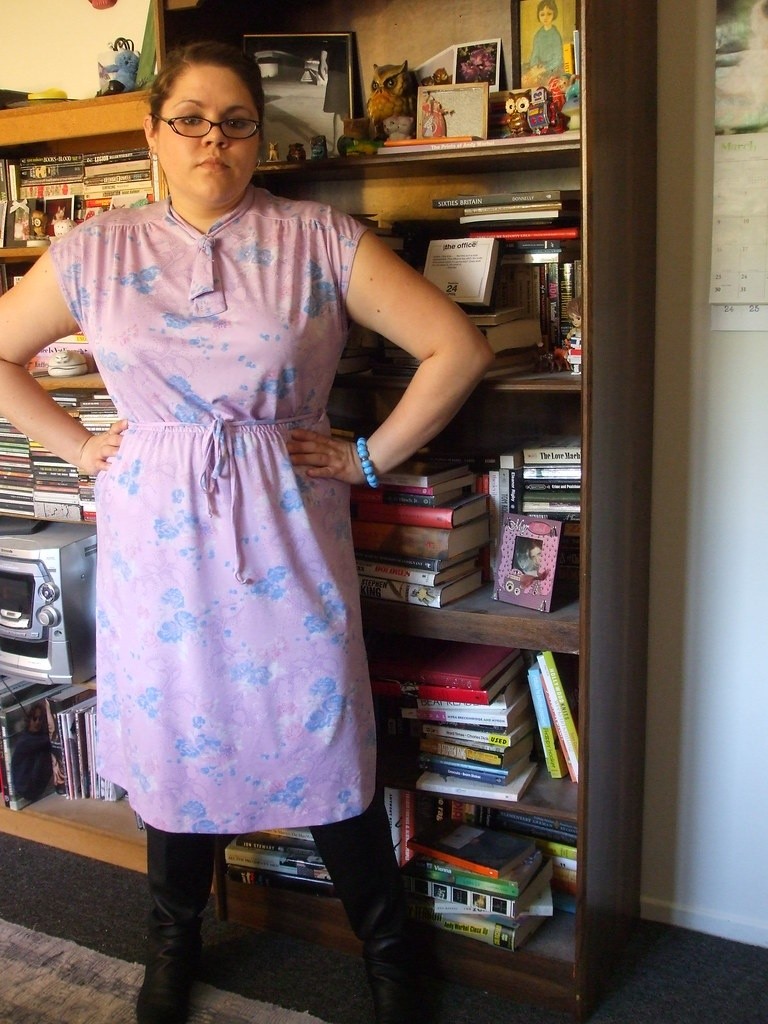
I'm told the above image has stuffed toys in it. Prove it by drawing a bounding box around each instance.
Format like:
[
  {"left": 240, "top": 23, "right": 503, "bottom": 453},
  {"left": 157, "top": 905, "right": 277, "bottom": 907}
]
[{"left": 103, "top": 50, "right": 139, "bottom": 93}]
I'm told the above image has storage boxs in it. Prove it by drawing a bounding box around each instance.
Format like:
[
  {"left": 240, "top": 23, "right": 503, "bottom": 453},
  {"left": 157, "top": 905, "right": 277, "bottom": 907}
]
[{"left": 6, "top": 198, "right": 37, "bottom": 247}]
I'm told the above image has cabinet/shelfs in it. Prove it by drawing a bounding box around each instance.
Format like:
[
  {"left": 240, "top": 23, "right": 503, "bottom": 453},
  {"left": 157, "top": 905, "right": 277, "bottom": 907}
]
[{"left": 155, "top": 0, "right": 659, "bottom": 1024}]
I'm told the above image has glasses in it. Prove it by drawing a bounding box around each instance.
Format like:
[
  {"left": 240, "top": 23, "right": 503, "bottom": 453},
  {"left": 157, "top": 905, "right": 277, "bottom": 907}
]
[{"left": 150, "top": 112, "right": 264, "bottom": 140}]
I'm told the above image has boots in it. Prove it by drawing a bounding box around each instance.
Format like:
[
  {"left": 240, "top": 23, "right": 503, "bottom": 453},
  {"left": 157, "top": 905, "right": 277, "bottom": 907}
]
[
  {"left": 311, "top": 787, "right": 430, "bottom": 1024},
  {"left": 137, "top": 823, "right": 214, "bottom": 1024}
]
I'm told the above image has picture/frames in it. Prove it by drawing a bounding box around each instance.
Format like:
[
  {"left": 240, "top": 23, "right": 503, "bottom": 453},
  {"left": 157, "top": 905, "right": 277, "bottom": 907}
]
[
  {"left": 417, "top": 82, "right": 489, "bottom": 139},
  {"left": 44, "top": 195, "right": 74, "bottom": 236},
  {"left": 452, "top": 36, "right": 500, "bottom": 91},
  {"left": 241, "top": 29, "right": 356, "bottom": 161},
  {"left": 490, "top": 512, "right": 563, "bottom": 612},
  {"left": 511, "top": 0, "right": 582, "bottom": 89}
]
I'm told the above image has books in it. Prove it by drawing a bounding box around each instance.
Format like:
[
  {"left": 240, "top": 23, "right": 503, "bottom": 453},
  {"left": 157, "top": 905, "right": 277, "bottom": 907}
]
[
  {"left": 0, "top": 677, "right": 146, "bottom": 832},
  {"left": 326, "top": 404, "right": 584, "bottom": 615},
  {"left": 0, "top": 147, "right": 155, "bottom": 248},
  {"left": 338, "top": 190, "right": 581, "bottom": 390},
  {"left": 563, "top": 43, "right": 575, "bottom": 85},
  {"left": 0, "top": 394, "right": 120, "bottom": 522},
  {"left": 222, "top": 635, "right": 579, "bottom": 952}
]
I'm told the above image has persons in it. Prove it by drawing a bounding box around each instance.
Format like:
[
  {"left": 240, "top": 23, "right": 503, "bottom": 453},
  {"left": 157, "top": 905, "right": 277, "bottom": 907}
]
[
  {"left": 567, "top": 296, "right": 581, "bottom": 370},
  {"left": 0, "top": 40, "right": 495, "bottom": 1024},
  {"left": 317, "top": 38, "right": 349, "bottom": 156},
  {"left": 11, "top": 704, "right": 51, "bottom": 802},
  {"left": 528, "top": 540, "right": 542, "bottom": 577}
]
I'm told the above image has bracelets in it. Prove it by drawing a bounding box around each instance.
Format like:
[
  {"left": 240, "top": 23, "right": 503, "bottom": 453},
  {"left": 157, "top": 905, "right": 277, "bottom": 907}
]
[{"left": 357, "top": 438, "right": 379, "bottom": 488}]
